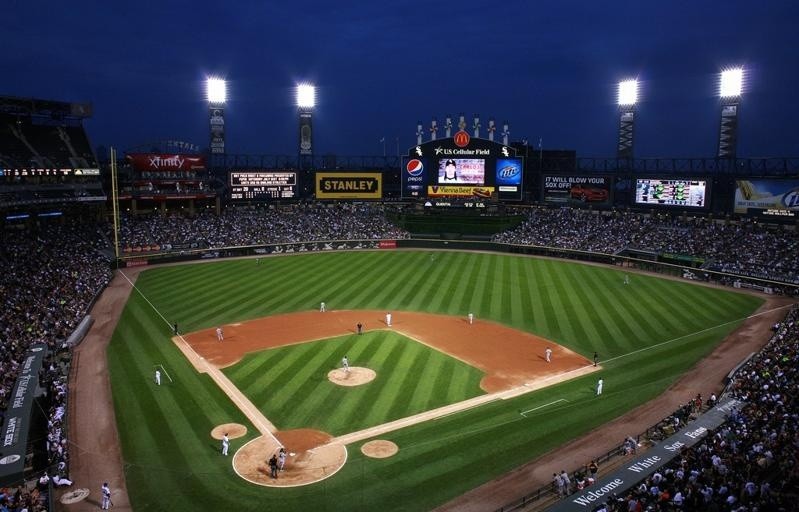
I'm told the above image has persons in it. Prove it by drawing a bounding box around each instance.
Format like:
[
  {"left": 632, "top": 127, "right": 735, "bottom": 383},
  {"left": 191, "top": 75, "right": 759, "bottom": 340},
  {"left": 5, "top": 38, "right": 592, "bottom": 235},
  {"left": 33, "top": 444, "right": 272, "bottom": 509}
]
[
  {"left": 467, "top": 312, "right": 473, "bottom": 324},
  {"left": 357, "top": 322, "right": 362, "bottom": 334},
  {"left": 386, "top": 312, "right": 392, "bottom": 326},
  {"left": 545, "top": 346, "right": 552, "bottom": 363},
  {"left": 155, "top": 368, "right": 161, "bottom": 386},
  {"left": 343, "top": 355, "right": 349, "bottom": 372},
  {"left": 174, "top": 322, "right": 178, "bottom": 335},
  {"left": 216, "top": 326, "right": 224, "bottom": 341},
  {"left": 320, "top": 300, "right": 325, "bottom": 312},
  {"left": 269, "top": 454, "right": 278, "bottom": 478},
  {"left": 277, "top": 448, "right": 288, "bottom": 472},
  {"left": 220, "top": 433, "right": 231, "bottom": 456}
]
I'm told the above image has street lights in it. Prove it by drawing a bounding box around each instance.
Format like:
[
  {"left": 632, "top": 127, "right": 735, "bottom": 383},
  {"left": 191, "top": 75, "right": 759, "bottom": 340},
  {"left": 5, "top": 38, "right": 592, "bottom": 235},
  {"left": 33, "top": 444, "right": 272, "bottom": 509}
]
[
  {"left": 295, "top": 82, "right": 316, "bottom": 172},
  {"left": 205, "top": 77, "right": 228, "bottom": 185},
  {"left": 616, "top": 79, "right": 639, "bottom": 155},
  {"left": 717, "top": 66, "right": 743, "bottom": 160}
]
